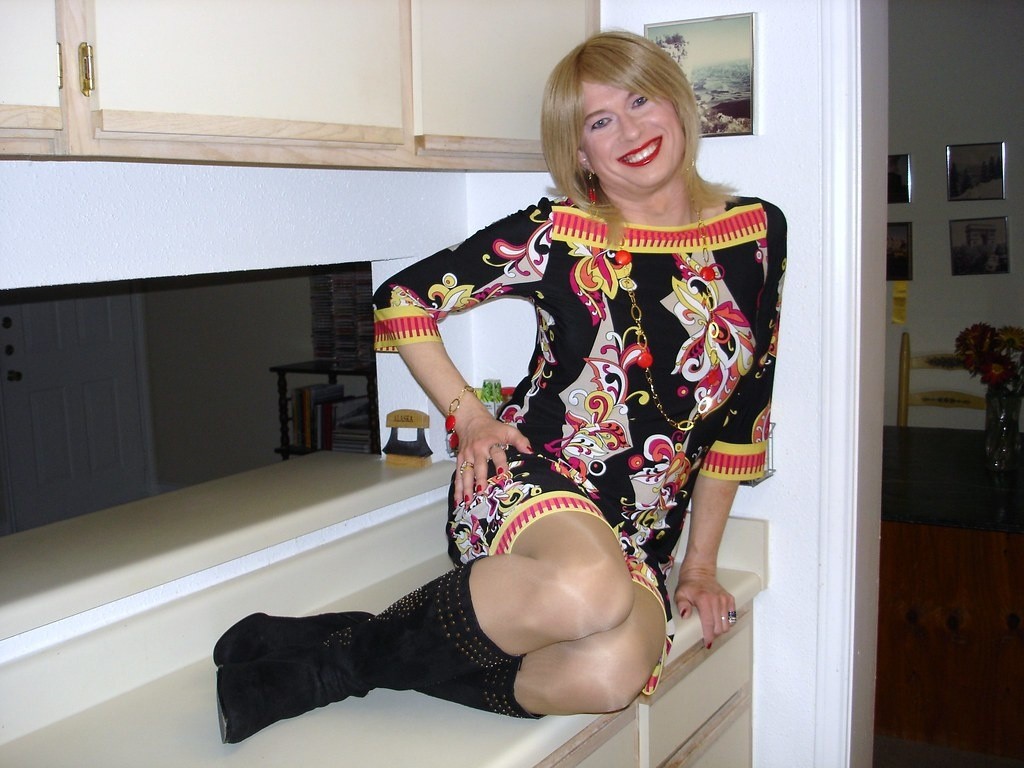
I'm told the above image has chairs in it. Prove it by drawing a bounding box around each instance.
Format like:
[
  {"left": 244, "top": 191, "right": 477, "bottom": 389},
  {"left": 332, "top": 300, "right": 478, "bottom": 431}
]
[{"left": 898, "top": 332, "right": 988, "bottom": 428}]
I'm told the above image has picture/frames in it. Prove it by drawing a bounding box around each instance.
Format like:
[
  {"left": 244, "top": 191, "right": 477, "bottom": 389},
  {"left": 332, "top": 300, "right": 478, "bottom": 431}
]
[
  {"left": 949, "top": 216, "right": 1010, "bottom": 276},
  {"left": 643, "top": 12, "right": 758, "bottom": 138},
  {"left": 888, "top": 154, "right": 912, "bottom": 205},
  {"left": 886, "top": 221, "right": 913, "bottom": 281},
  {"left": 946, "top": 142, "right": 1006, "bottom": 201}
]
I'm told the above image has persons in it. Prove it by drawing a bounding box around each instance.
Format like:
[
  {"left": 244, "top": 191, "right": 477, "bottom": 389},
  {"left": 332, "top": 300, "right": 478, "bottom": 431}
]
[{"left": 212, "top": 27, "right": 789, "bottom": 749}]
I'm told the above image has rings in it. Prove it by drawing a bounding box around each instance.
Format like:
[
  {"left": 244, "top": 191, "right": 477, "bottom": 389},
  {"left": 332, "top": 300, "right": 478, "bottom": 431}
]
[
  {"left": 489, "top": 442, "right": 510, "bottom": 454},
  {"left": 721, "top": 617, "right": 728, "bottom": 620},
  {"left": 460, "top": 460, "right": 474, "bottom": 474},
  {"left": 728, "top": 611, "right": 737, "bottom": 623}
]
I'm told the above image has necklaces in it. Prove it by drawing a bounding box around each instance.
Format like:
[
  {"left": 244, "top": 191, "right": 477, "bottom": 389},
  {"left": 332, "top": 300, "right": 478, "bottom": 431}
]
[{"left": 619, "top": 198, "right": 719, "bottom": 433}]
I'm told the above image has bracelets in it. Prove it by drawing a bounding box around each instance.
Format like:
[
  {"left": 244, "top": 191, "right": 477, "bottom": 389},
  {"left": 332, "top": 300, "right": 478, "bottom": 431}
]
[{"left": 445, "top": 386, "right": 478, "bottom": 458}]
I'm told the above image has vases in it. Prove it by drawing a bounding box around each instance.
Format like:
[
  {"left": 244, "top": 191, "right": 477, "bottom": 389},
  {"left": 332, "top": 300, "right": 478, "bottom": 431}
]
[{"left": 984, "top": 393, "right": 1022, "bottom": 470}]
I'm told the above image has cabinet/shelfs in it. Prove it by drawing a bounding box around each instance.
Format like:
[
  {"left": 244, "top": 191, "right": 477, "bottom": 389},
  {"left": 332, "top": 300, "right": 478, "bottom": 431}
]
[
  {"left": 1, "top": 546, "right": 755, "bottom": 768},
  {"left": 269, "top": 358, "right": 380, "bottom": 461},
  {"left": 0, "top": 0, "right": 601, "bottom": 173}
]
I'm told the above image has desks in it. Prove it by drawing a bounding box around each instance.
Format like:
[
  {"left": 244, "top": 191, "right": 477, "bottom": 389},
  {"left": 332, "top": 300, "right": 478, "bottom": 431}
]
[{"left": 875, "top": 427, "right": 1024, "bottom": 760}]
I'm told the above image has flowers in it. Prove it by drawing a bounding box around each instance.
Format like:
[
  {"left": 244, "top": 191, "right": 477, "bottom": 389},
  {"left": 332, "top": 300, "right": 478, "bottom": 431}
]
[{"left": 954, "top": 322, "right": 1024, "bottom": 466}]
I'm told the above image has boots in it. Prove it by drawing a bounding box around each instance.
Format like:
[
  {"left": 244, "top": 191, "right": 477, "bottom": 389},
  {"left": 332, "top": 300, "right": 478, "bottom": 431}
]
[
  {"left": 214, "top": 556, "right": 524, "bottom": 745},
  {"left": 213, "top": 612, "right": 549, "bottom": 718}
]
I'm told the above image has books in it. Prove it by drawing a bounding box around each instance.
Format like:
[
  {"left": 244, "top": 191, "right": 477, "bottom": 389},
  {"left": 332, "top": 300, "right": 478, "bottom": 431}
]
[{"left": 285, "top": 384, "right": 373, "bottom": 453}]
